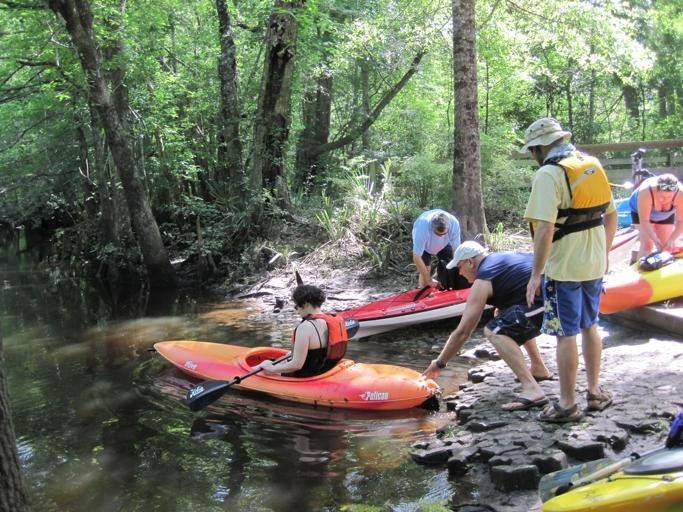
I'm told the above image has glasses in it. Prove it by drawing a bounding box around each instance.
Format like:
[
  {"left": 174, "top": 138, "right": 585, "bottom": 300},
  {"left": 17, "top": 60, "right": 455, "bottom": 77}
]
[
  {"left": 294, "top": 304, "right": 298, "bottom": 310},
  {"left": 434, "top": 233, "right": 446, "bottom": 236},
  {"left": 659, "top": 184, "right": 677, "bottom": 192}
]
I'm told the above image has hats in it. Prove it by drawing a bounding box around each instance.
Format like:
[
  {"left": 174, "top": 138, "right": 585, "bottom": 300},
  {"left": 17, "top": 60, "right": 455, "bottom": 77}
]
[
  {"left": 519, "top": 117, "right": 571, "bottom": 153},
  {"left": 445, "top": 240, "right": 485, "bottom": 270}
]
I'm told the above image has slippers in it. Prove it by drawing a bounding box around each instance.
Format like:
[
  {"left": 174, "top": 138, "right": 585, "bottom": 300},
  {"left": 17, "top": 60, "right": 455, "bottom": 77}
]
[
  {"left": 514, "top": 372, "right": 553, "bottom": 382},
  {"left": 501, "top": 396, "right": 548, "bottom": 411}
]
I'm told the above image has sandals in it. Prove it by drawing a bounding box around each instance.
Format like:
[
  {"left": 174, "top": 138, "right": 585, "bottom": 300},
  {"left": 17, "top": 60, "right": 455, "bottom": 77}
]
[
  {"left": 536, "top": 402, "right": 583, "bottom": 422},
  {"left": 586, "top": 389, "right": 612, "bottom": 411}
]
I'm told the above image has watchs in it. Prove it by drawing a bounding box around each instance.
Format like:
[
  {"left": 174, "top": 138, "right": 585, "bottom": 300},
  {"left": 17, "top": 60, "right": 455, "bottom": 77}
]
[{"left": 433, "top": 358, "right": 447, "bottom": 369}]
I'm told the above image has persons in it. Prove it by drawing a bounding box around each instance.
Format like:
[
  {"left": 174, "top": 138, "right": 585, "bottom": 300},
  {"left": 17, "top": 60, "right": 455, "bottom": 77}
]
[
  {"left": 627, "top": 173, "right": 682, "bottom": 262},
  {"left": 258, "top": 284, "right": 346, "bottom": 379},
  {"left": 409, "top": 208, "right": 463, "bottom": 292},
  {"left": 520, "top": 117, "right": 618, "bottom": 425},
  {"left": 630, "top": 147, "right": 655, "bottom": 190},
  {"left": 421, "top": 240, "right": 556, "bottom": 412},
  {"left": 222, "top": 419, "right": 333, "bottom": 511}
]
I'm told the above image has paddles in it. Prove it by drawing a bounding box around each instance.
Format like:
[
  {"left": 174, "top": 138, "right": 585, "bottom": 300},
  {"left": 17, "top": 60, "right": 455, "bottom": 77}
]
[
  {"left": 186, "top": 316, "right": 360, "bottom": 413},
  {"left": 539, "top": 446, "right": 664, "bottom": 505}
]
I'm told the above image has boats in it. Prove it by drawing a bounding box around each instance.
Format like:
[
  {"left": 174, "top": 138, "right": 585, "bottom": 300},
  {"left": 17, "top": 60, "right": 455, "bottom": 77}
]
[
  {"left": 578, "top": 246, "right": 681, "bottom": 314},
  {"left": 301, "top": 224, "right": 642, "bottom": 325},
  {"left": 518, "top": 434, "right": 682, "bottom": 510},
  {"left": 143, "top": 333, "right": 441, "bottom": 418},
  {"left": 579, "top": 176, "right": 676, "bottom": 234}
]
